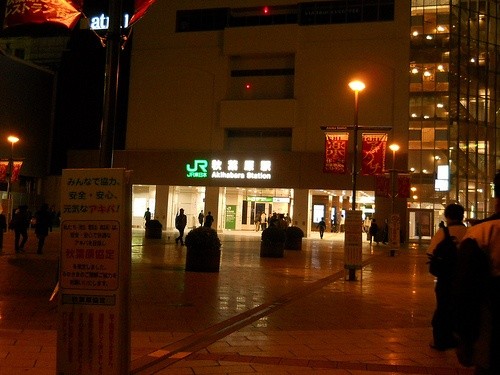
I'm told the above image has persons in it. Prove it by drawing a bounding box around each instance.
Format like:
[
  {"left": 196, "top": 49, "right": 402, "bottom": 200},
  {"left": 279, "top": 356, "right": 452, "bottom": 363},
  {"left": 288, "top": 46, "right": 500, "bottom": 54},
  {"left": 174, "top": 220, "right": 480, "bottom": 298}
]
[
  {"left": 429, "top": 171, "right": 500, "bottom": 374},
  {"left": 370, "top": 217, "right": 389, "bottom": 247},
  {"left": 317, "top": 217, "right": 326, "bottom": 238},
  {"left": 144, "top": 206, "right": 217, "bottom": 247},
  {"left": 0, "top": 200, "right": 57, "bottom": 254},
  {"left": 253, "top": 210, "right": 291, "bottom": 228}
]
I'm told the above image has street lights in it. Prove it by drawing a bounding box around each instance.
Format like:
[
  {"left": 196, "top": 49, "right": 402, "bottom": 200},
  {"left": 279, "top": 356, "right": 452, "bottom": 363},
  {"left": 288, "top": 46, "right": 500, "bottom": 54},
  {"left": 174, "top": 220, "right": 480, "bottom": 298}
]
[
  {"left": 8, "top": 134, "right": 19, "bottom": 183},
  {"left": 346, "top": 78, "right": 369, "bottom": 282},
  {"left": 389, "top": 143, "right": 400, "bottom": 257}
]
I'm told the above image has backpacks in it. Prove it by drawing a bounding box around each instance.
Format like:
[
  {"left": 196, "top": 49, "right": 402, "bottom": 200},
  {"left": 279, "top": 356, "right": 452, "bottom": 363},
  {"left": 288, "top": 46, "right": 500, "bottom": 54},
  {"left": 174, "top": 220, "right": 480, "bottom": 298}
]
[{"left": 430, "top": 227, "right": 458, "bottom": 279}]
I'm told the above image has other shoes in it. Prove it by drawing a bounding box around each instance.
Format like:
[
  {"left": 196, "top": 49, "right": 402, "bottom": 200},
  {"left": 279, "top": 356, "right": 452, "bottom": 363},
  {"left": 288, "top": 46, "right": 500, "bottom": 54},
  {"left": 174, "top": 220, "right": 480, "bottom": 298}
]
[
  {"left": 181, "top": 242, "right": 184, "bottom": 246},
  {"left": 175, "top": 238, "right": 179, "bottom": 245},
  {"left": 12, "top": 241, "right": 49, "bottom": 256}
]
